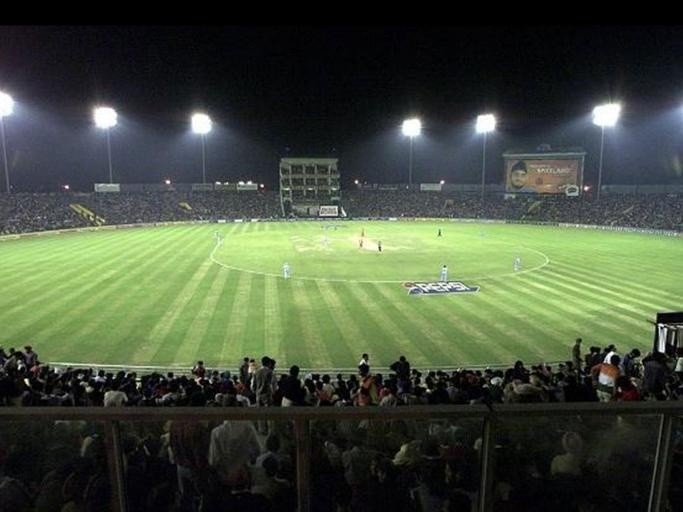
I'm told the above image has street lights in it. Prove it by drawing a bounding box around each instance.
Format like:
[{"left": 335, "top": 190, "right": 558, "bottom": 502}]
[
  {"left": 1, "top": 91, "right": 15, "bottom": 197},
  {"left": 401, "top": 117, "right": 422, "bottom": 185},
  {"left": 93, "top": 106, "right": 117, "bottom": 183},
  {"left": 476, "top": 114, "right": 496, "bottom": 199},
  {"left": 191, "top": 113, "right": 213, "bottom": 184},
  {"left": 592, "top": 102, "right": 622, "bottom": 198}
]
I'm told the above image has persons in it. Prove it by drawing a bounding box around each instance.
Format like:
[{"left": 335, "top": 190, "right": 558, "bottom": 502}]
[
  {"left": 279, "top": 262, "right": 290, "bottom": 279},
  {"left": 512, "top": 255, "right": 521, "bottom": 273},
  {"left": 507, "top": 160, "right": 537, "bottom": 193},
  {"left": 359, "top": 238, "right": 364, "bottom": 250},
  {"left": 0, "top": 337, "right": 682, "bottom": 510},
  {"left": 0, "top": 182, "right": 683, "bottom": 237},
  {"left": 436, "top": 228, "right": 443, "bottom": 237},
  {"left": 378, "top": 240, "right": 382, "bottom": 254},
  {"left": 439, "top": 265, "right": 448, "bottom": 283}
]
